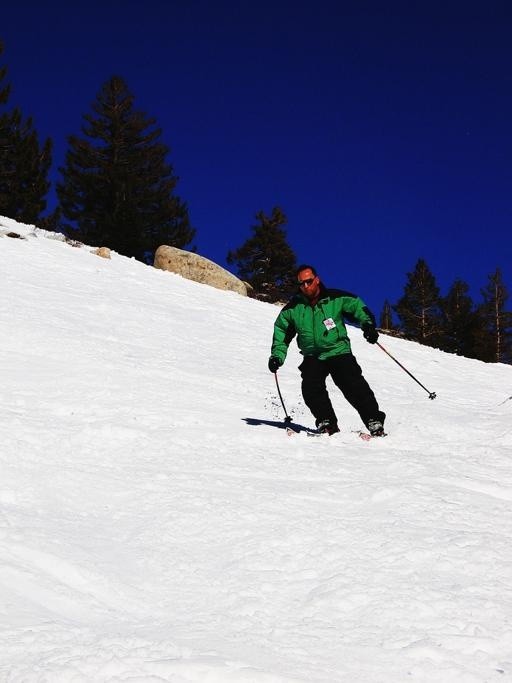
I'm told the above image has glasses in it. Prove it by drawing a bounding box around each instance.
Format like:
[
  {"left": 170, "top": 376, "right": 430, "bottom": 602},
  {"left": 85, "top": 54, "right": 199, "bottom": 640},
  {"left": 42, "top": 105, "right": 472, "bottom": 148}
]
[{"left": 296, "top": 276, "right": 317, "bottom": 288}]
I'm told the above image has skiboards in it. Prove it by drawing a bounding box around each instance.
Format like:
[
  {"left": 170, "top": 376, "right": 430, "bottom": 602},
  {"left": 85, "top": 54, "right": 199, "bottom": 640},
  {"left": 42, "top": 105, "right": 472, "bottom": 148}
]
[{"left": 286, "top": 427, "right": 388, "bottom": 442}]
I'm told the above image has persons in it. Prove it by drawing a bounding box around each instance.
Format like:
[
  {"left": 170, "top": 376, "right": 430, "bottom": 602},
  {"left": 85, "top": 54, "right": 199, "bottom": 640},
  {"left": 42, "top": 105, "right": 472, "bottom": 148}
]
[{"left": 264, "top": 262, "right": 386, "bottom": 435}]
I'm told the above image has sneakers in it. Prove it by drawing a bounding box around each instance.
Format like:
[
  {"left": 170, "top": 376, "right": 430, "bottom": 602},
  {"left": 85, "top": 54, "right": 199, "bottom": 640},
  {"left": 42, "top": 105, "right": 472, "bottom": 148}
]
[
  {"left": 368, "top": 420, "right": 386, "bottom": 437},
  {"left": 306, "top": 420, "right": 338, "bottom": 436}
]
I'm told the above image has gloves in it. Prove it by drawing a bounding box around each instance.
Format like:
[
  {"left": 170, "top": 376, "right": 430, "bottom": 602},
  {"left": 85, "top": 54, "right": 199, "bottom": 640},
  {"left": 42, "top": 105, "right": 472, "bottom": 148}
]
[
  {"left": 268, "top": 357, "right": 280, "bottom": 373},
  {"left": 363, "top": 323, "right": 379, "bottom": 344}
]
